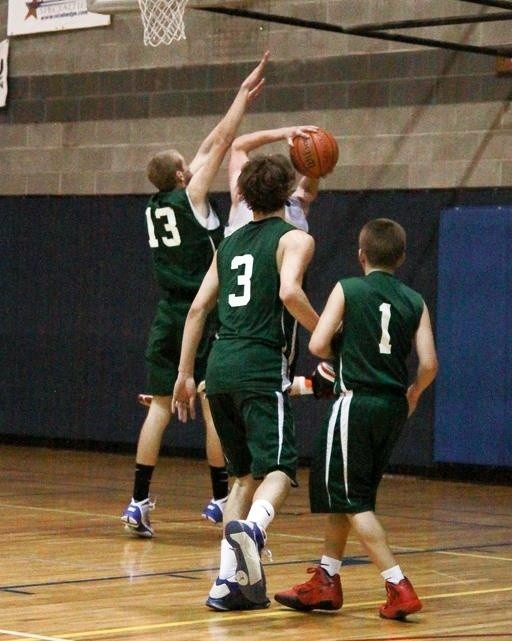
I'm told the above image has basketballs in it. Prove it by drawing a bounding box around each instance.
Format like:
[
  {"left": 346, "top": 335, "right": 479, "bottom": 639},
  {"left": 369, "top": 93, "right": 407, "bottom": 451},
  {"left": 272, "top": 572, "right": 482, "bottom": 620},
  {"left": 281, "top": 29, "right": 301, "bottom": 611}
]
[{"left": 289, "top": 128, "right": 338, "bottom": 177}]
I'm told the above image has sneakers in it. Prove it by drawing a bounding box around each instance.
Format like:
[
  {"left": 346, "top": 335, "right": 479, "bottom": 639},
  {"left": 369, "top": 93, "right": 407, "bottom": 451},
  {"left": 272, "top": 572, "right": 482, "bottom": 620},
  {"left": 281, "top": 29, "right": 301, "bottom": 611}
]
[
  {"left": 207, "top": 518, "right": 271, "bottom": 609},
  {"left": 274, "top": 564, "right": 344, "bottom": 611},
  {"left": 202, "top": 498, "right": 226, "bottom": 522},
  {"left": 378, "top": 576, "right": 422, "bottom": 619},
  {"left": 121, "top": 496, "right": 157, "bottom": 539}
]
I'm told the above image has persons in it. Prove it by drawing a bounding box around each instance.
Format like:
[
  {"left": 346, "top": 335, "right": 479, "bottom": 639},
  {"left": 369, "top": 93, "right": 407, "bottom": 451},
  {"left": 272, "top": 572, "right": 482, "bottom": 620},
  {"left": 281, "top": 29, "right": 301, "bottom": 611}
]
[
  {"left": 273, "top": 216, "right": 440, "bottom": 618},
  {"left": 170, "top": 126, "right": 321, "bottom": 616},
  {"left": 122, "top": 50, "right": 269, "bottom": 540}
]
